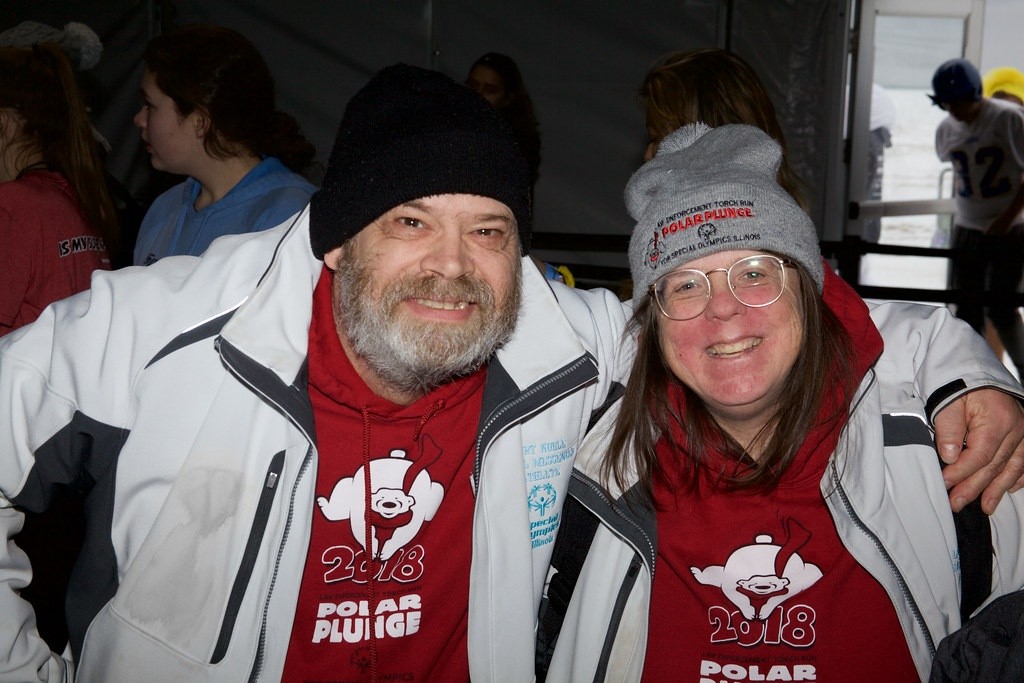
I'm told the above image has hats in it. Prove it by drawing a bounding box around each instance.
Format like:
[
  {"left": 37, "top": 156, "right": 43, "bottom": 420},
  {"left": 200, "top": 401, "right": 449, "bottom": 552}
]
[
  {"left": 622, "top": 123, "right": 828, "bottom": 320},
  {"left": 933, "top": 59, "right": 985, "bottom": 105},
  {"left": 983, "top": 68, "right": 1024, "bottom": 104},
  {"left": 309, "top": 63, "right": 533, "bottom": 259}
]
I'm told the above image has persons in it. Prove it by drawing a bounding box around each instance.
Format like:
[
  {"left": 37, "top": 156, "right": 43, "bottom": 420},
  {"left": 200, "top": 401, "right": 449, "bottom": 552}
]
[
  {"left": 976, "top": 66, "right": 1023, "bottom": 360},
  {"left": 463, "top": 54, "right": 540, "bottom": 238},
  {"left": 128, "top": 27, "right": 320, "bottom": 265},
  {"left": 0, "top": 20, "right": 132, "bottom": 268},
  {"left": 637, "top": 47, "right": 811, "bottom": 224},
  {"left": 928, "top": 56, "right": 1022, "bottom": 387},
  {"left": 1, "top": 44, "right": 115, "bottom": 338},
  {"left": 528, "top": 123, "right": 1023, "bottom": 682},
  {"left": 1, "top": 65, "right": 1024, "bottom": 683}
]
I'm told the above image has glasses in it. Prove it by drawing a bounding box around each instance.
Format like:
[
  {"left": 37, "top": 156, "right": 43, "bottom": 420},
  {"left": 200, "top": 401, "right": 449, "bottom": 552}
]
[{"left": 647, "top": 254, "right": 802, "bottom": 320}]
